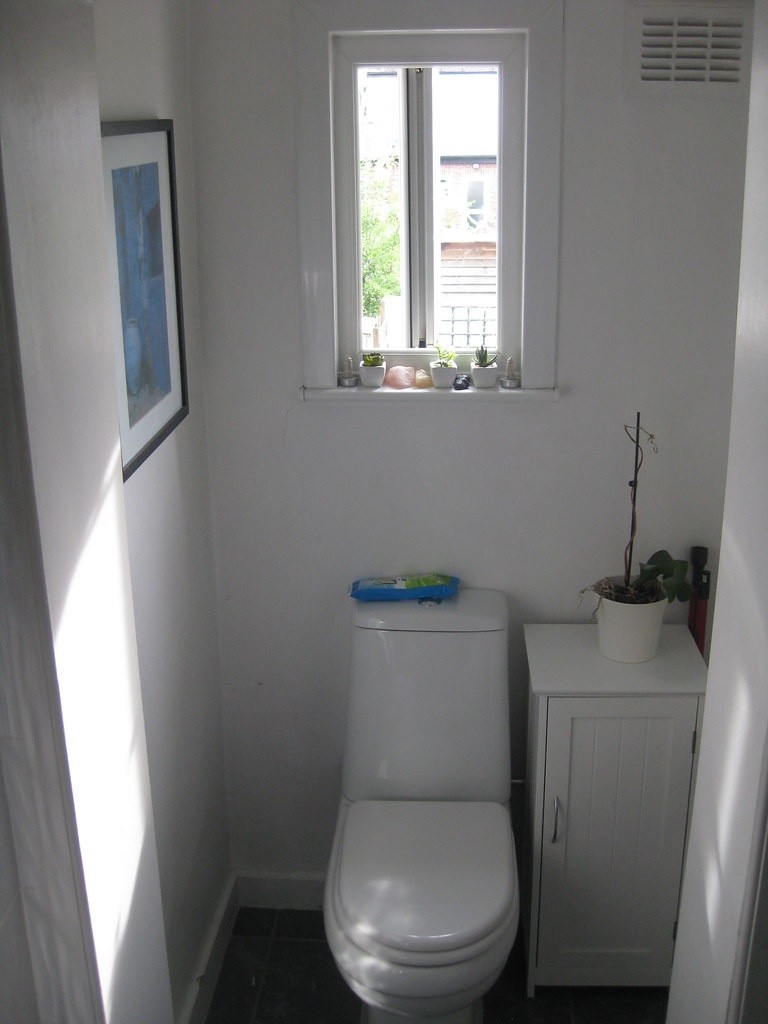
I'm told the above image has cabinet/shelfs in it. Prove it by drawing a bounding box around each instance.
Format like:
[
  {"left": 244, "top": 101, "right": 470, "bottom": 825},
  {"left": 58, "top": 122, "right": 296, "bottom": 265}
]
[{"left": 522, "top": 623, "right": 708, "bottom": 998}]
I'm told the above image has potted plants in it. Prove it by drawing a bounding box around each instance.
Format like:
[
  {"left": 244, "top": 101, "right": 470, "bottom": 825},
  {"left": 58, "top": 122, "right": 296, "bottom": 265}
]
[
  {"left": 430, "top": 340, "right": 457, "bottom": 388},
  {"left": 470, "top": 345, "right": 498, "bottom": 388},
  {"left": 359, "top": 352, "right": 386, "bottom": 388},
  {"left": 578, "top": 412, "right": 711, "bottom": 664}
]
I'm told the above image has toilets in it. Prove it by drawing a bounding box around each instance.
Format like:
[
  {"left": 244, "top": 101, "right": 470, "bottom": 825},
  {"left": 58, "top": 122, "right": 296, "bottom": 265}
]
[{"left": 323, "top": 587, "right": 520, "bottom": 1024}]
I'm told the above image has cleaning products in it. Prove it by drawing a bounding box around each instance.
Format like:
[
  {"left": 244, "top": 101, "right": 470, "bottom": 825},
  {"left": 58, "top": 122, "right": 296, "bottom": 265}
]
[{"left": 348, "top": 573, "right": 459, "bottom": 601}]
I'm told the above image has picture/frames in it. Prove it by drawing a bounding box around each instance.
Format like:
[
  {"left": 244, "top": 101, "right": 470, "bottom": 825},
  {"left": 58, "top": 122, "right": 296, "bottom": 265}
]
[{"left": 100, "top": 118, "right": 190, "bottom": 484}]
